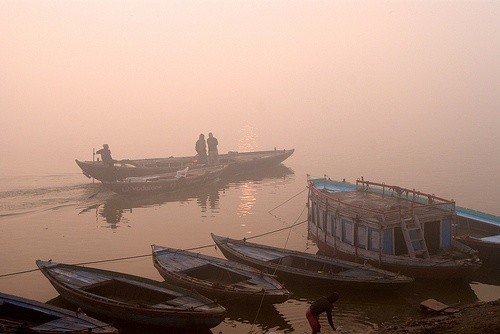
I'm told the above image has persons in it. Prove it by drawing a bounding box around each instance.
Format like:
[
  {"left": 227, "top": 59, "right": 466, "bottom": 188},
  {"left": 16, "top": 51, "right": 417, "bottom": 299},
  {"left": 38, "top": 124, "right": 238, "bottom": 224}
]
[
  {"left": 96, "top": 144, "right": 114, "bottom": 167},
  {"left": 195, "top": 134, "right": 208, "bottom": 164},
  {"left": 207, "top": 132, "right": 218, "bottom": 165},
  {"left": 306, "top": 293, "right": 338, "bottom": 334}
]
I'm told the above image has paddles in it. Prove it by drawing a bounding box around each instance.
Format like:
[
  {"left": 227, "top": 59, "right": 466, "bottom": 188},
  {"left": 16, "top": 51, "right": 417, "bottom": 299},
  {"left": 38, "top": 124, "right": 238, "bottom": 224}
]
[{"left": 90, "top": 147, "right": 97, "bottom": 184}]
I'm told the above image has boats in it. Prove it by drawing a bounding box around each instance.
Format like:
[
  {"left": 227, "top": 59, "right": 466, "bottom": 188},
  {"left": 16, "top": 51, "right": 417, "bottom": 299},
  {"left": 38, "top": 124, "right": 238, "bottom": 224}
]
[
  {"left": 35, "top": 259, "right": 228, "bottom": 332},
  {"left": 209, "top": 232, "right": 415, "bottom": 292},
  {"left": 101, "top": 164, "right": 227, "bottom": 196},
  {"left": 150, "top": 243, "right": 290, "bottom": 305},
  {"left": 307, "top": 174, "right": 500, "bottom": 254},
  {"left": 74, "top": 149, "right": 295, "bottom": 185},
  {"left": 0, "top": 291, "right": 119, "bottom": 334},
  {"left": 305, "top": 180, "right": 485, "bottom": 288}
]
